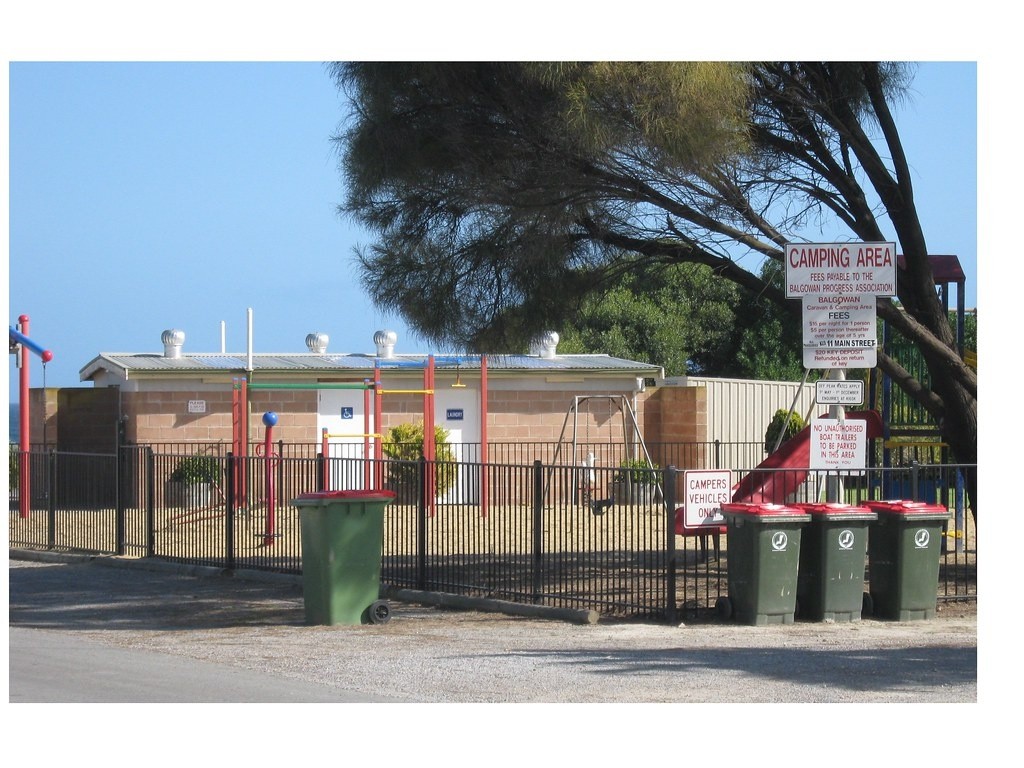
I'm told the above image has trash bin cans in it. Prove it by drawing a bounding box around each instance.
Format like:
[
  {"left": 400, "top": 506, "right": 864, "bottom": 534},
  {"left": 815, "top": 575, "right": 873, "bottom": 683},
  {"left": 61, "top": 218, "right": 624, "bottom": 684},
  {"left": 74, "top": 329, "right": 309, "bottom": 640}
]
[
  {"left": 860, "top": 500, "right": 951, "bottom": 622},
  {"left": 786, "top": 502, "right": 878, "bottom": 621},
  {"left": 290, "top": 490, "right": 397, "bottom": 626},
  {"left": 715, "top": 502, "right": 812, "bottom": 626}
]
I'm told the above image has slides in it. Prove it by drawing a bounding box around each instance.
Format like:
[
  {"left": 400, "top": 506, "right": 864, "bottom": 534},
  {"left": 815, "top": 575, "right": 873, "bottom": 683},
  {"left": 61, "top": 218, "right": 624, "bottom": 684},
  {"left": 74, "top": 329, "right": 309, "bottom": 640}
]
[{"left": 674, "top": 409, "right": 884, "bottom": 535}]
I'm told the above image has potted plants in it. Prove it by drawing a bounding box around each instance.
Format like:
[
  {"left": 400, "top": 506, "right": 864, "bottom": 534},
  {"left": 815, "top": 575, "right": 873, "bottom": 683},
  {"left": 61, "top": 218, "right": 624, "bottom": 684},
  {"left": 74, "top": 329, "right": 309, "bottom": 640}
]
[
  {"left": 614, "top": 458, "right": 663, "bottom": 506},
  {"left": 169, "top": 452, "right": 223, "bottom": 507}
]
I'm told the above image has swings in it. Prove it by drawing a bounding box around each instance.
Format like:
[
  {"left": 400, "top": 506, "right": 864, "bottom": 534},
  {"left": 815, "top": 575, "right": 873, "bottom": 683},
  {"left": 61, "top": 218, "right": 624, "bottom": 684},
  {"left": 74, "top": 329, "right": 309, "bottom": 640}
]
[{"left": 586, "top": 398, "right": 615, "bottom": 515}]
